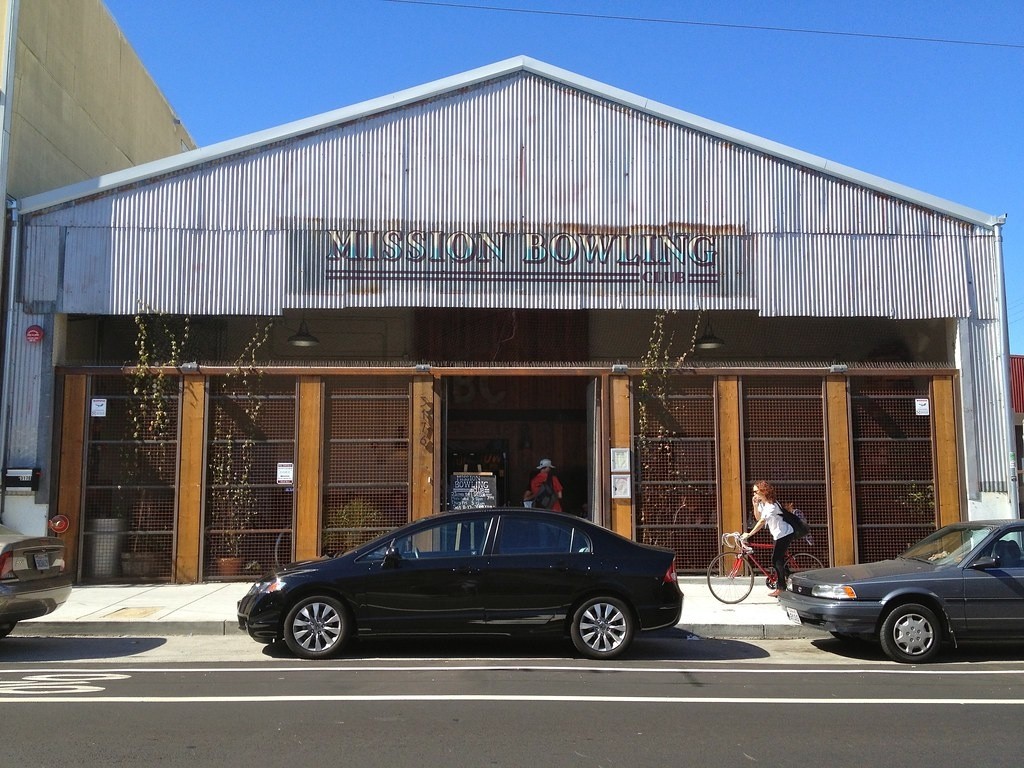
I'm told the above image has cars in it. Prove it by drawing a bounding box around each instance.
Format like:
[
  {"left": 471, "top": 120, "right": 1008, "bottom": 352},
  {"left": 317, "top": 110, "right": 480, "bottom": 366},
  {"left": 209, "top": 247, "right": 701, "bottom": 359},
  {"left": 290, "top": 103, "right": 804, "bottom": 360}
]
[
  {"left": 775, "top": 518, "right": 1024, "bottom": 665},
  {"left": 0, "top": 523, "right": 75, "bottom": 639},
  {"left": 232, "top": 506, "right": 687, "bottom": 661}
]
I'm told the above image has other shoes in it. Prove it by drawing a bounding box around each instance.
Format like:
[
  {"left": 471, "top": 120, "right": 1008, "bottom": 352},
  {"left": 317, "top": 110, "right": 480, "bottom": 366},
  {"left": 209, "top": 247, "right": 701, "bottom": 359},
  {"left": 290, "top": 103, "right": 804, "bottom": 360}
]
[{"left": 768, "top": 589, "right": 786, "bottom": 597}]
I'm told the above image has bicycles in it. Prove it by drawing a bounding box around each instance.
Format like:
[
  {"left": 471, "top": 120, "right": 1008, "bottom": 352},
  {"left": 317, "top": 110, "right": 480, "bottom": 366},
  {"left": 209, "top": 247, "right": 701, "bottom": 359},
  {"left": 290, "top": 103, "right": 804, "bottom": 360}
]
[{"left": 707, "top": 531, "right": 825, "bottom": 605}]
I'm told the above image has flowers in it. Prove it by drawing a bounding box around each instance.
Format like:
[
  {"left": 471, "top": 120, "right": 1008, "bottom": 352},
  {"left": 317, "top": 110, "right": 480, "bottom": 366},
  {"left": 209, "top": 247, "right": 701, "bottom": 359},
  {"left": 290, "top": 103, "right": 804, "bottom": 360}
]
[
  {"left": 122, "top": 291, "right": 192, "bottom": 551},
  {"left": 208, "top": 312, "right": 276, "bottom": 554}
]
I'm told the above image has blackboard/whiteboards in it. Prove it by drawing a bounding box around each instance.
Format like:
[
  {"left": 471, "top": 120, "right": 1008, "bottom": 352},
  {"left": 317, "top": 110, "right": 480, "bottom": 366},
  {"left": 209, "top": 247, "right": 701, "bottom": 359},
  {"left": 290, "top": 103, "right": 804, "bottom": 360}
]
[{"left": 449, "top": 475, "right": 496, "bottom": 512}]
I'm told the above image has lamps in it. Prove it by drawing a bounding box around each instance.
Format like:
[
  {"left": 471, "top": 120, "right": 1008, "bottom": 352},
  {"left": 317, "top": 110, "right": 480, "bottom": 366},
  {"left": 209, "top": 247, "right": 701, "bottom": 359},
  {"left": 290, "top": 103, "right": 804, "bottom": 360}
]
[
  {"left": 694, "top": 311, "right": 725, "bottom": 349},
  {"left": 287, "top": 312, "right": 319, "bottom": 347}
]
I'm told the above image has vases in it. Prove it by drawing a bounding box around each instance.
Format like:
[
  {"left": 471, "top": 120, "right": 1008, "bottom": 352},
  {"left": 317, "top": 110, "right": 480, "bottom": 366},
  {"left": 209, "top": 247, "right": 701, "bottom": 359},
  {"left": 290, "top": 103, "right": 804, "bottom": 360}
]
[
  {"left": 220, "top": 557, "right": 244, "bottom": 576},
  {"left": 88, "top": 517, "right": 124, "bottom": 580},
  {"left": 124, "top": 552, "right": 160, "bottom": 575}
]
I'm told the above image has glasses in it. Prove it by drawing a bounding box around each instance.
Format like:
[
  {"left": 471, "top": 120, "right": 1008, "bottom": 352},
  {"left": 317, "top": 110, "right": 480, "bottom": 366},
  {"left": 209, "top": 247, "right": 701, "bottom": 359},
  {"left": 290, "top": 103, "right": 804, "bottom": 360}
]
[{"left": 752, "top": 491, "right": 757, "bottom": 494}]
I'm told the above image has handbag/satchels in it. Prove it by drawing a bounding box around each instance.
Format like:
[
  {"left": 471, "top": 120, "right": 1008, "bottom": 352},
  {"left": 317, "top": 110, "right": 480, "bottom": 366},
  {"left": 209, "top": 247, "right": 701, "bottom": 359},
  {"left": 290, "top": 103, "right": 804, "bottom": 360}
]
[{"left": 534, "top": 472, "right": 552, "bottom": 508}]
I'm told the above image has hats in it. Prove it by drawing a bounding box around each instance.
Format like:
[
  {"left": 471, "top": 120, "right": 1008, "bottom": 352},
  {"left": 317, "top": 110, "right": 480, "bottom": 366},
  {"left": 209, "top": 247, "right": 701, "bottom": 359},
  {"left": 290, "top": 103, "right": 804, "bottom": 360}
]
[{"left": 536, "top": 459, "right": 555, "bottom": 469}]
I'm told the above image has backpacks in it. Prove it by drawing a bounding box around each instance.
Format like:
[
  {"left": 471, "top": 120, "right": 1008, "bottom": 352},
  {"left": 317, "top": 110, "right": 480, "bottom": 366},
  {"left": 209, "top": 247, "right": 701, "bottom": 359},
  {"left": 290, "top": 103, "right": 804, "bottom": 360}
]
[{"left": 774, "top": 501, "right": 809, "bottom": 539}]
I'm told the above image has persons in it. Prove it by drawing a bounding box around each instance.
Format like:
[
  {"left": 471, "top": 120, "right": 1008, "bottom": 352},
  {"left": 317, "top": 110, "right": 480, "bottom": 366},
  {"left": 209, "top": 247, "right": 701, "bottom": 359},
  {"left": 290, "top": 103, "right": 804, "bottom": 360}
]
[
  {"left": 740, "top": 481, "right": 794, "bottom": 596},
  {"left": 647, "top": 491, "right": 703, "bottom": 548},
  {"left": 524, "top": 459, "right": 563, "bottom": 546},
  {"left": 781, "top": 496, "right": 813, "bottom": 567}
]
